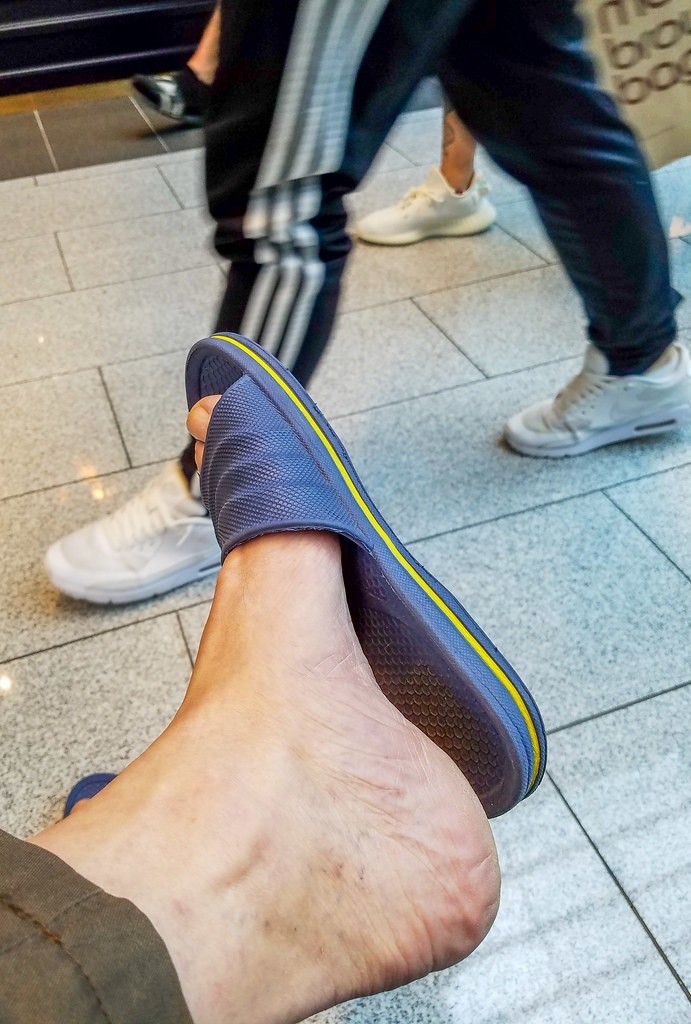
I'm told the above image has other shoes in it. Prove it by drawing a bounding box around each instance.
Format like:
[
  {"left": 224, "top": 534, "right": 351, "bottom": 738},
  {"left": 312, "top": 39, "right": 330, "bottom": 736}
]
[{"left": 131, "top": 70, "right": 208, "bottom": 126}]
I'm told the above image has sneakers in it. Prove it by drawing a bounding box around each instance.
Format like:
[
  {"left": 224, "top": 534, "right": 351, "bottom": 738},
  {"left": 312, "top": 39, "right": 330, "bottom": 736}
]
[
  {"left": 503, "top": 341, "right": 691, "bottom": 458},
  {"left": 357, "top": 166, "right": 497, "bottom": 246},
  {"left": 43, "top": 458, "right": 222, "bottom": 604}
]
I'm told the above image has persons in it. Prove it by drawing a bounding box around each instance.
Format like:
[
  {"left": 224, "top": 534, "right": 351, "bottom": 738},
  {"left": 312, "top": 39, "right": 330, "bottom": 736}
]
[
  {"left": 0, "top": 330, "right": 544, "bottom": 1024},
  {"left": 45, "top": 0, "right": 691, "bottom": 607}
]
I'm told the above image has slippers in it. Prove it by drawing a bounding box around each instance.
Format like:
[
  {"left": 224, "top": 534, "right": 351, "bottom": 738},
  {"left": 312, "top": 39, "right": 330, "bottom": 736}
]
[
  {"left": 63, "top": 772, "right": 117, "bottom": 817},
  {"left": 184, "top": 332, "right": 548, "bottom": 820}
]
